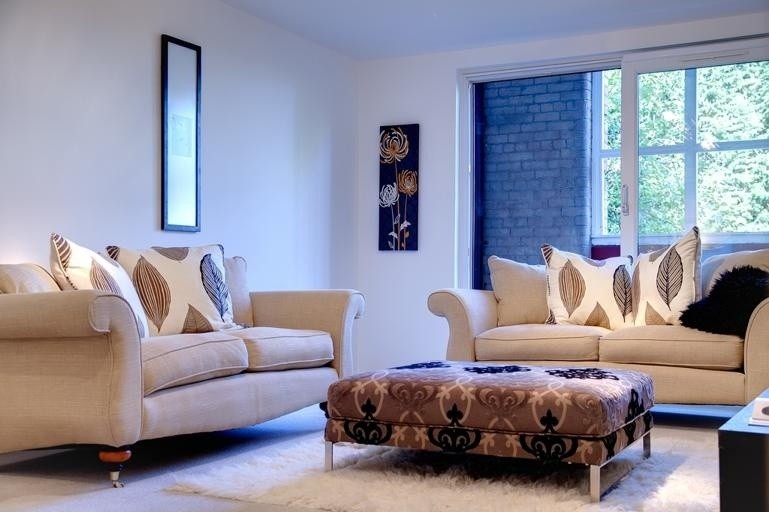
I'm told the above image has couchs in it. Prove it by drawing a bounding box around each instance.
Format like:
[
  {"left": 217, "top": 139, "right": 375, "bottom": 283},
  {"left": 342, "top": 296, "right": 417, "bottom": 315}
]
[
  {"left": 0, "top": 255, "right": 364, "bottom": 489},
  {"left": 50, "top": 232, "right": 243, "bottom": 336}
]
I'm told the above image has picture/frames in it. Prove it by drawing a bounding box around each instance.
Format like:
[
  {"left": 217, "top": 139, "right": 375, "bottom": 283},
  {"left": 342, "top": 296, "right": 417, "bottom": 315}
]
[{"left": 159, "top": 35, "right": 203, "bottom": 233}]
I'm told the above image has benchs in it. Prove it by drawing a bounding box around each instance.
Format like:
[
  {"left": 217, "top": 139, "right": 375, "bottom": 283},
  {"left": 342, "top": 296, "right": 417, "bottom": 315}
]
[{"left": 322, "top": 360, "right": 654, "bottom": 504}]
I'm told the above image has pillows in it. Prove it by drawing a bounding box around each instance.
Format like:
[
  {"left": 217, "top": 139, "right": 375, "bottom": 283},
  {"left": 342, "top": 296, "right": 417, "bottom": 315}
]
[{"left": 542, "top": 226, "right": 703, "bottom": 330}]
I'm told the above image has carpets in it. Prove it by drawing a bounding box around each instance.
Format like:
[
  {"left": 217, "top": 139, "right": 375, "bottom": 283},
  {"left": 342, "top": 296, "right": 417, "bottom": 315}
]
[{"left": 160, "top": 417, "right": 726, "bottom": 512}]
